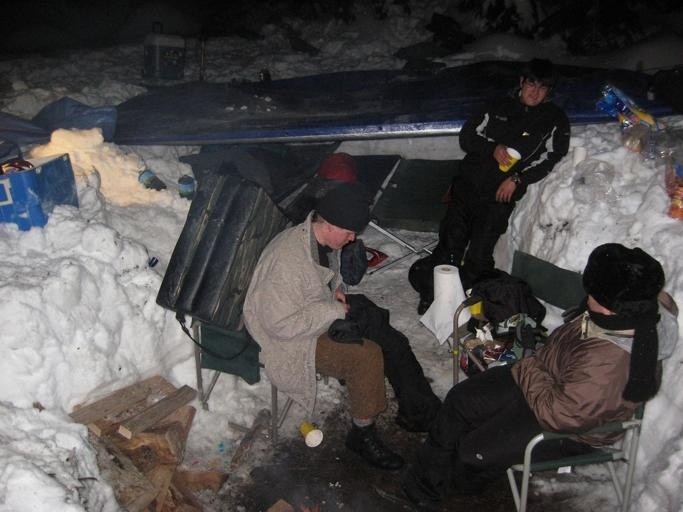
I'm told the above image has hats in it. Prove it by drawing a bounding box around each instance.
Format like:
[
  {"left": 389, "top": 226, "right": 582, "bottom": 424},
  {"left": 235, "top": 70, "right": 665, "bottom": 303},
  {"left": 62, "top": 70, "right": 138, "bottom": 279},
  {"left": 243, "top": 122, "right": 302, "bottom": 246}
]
[
  {"left": 583, "top": 243, "right": 664, "bottom": 313},
  {"left": 317, "top": 182, "right": 369, "bottom": 233}
]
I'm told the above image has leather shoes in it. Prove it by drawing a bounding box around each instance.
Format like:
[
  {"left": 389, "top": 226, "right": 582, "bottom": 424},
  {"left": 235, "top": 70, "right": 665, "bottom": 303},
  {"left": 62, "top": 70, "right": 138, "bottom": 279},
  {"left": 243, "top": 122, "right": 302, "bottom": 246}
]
[{"left": 346, "top": 424, "right": 405, "bottom": 469}]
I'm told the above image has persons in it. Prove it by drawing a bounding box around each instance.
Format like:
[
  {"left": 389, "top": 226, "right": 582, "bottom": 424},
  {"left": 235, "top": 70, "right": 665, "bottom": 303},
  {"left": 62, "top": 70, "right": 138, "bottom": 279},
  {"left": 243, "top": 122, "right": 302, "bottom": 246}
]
[
  {"left": 429, "top": 54, "right": 575, "bottom": 284},
  {"left": 372, "top": 240, "right": 683, "bottom": 512},
  {"left": 237, "top": 179, "right": 446, "bottom": 472}
]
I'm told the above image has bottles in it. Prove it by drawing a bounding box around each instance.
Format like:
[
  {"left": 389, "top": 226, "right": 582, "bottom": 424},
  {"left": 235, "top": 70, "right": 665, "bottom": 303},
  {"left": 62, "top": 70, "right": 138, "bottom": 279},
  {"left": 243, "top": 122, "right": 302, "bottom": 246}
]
[
  {"left": 137, "top": 169, "right": 167, "bottom": 192},
  {"left": 178, "top": 174, "right": 195, "bottom": 200}
]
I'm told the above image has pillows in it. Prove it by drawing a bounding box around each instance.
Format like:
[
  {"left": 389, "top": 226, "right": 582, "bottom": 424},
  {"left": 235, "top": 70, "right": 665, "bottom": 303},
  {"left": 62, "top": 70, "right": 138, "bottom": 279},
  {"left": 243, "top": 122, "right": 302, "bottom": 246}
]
[{"left": 155, "top": 169, "right": 294, "bottom": 330}]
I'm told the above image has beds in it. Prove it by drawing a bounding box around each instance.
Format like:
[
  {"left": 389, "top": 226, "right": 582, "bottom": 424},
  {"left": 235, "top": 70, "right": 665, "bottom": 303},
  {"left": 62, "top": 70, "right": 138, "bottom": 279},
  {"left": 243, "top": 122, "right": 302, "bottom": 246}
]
[{"left": 113, "top": 62, "right": 683, "bottom": 146}]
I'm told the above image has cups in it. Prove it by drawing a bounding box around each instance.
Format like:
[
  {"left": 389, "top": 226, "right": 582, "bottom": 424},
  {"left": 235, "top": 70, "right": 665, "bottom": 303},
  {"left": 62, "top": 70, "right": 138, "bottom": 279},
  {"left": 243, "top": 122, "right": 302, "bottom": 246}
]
[
  {"left": 466, "top": 289, "right": 482, "bottom": 315},
  {"left": 299, "top": 421, "right": 324, "bottom": 448},
  {"left": 499, "top": 147, "right": 522, "bottom": 173},
  {"left": 572, "top": 146, "right": 588, "bottom": 167}
]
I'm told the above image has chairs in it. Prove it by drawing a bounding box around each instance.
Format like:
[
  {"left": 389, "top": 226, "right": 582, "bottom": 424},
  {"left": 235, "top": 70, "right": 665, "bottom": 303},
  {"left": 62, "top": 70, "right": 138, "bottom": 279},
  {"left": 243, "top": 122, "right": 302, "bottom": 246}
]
[
  {"left": 453, "top": 250, "right": 587, "bottom": 388},
  {"left": 189, "top": 316, "right": 329, "bottom": 448},
  {"left": 506, "top": 356, "right": 646, "bottom": 511}
]
